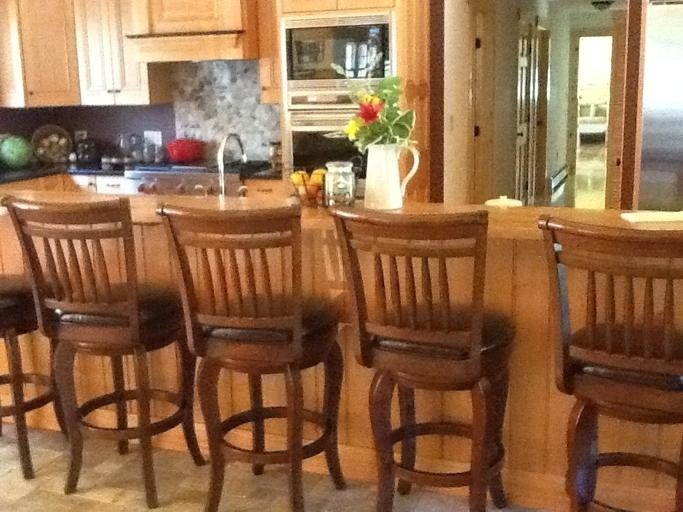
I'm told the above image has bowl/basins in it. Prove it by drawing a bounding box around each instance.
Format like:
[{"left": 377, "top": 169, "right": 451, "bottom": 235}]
[{"left": 168, "top": 139, "right": 205, "bottom": 163}]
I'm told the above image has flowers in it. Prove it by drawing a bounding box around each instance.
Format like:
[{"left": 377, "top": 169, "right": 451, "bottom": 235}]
[{"left": 342, "top": 73, "right": 417, "bottom": 157}]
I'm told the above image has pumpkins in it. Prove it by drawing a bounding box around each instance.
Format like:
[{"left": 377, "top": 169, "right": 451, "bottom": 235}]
[{"left": 0, "top": 134, "right": 32, "bottom": 170}]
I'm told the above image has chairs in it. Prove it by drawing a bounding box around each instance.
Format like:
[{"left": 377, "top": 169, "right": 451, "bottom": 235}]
[
  {"left": 154, "top": 200, "right": 349, "bottom": 512},
  {"left": 0, "top": 191, "right": 206, "bottom": 495},
  {"left": 0, "top": 271, "right": 68, "bottom": 480},
  {"left": 326, "top": 203, "right": 517, "bottom": 512},
  {"left": 537, "top": 214, "right": 683, "bottom": 512}
]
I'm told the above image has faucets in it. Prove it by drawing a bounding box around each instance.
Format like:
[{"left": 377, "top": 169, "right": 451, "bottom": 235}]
[{"left": 216, "top": 129, "right": 248, "bottom": 197}]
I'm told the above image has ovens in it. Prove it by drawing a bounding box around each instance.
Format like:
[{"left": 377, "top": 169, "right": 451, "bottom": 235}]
[{"left": 291, "top": 112, "right": 369, "bottom": 193}]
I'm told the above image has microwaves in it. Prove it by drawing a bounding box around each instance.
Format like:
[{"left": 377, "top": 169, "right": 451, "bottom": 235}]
[{"left": 285, "top": 23, "right": 393, "bottom": 106}]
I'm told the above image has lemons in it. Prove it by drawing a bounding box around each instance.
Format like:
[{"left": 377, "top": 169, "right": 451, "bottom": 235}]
[{"left": 291, "top": 168, "right": 325, "bottom": 185}]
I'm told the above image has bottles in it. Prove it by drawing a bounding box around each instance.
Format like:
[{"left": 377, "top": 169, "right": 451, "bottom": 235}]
[{"left": 269, "top": 141, "right": 283, "bottom": 173}]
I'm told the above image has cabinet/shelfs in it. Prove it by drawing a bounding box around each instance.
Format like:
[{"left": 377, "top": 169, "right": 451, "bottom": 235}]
[
  {"left": 72, "top": 2, "right": 150, "bottom": 109},
  {"left": 280, "top": 3, "right": 396, "bottom": 15},
  {"left": 2, "top": 1, "right": 81, "bottom": 109}
]
